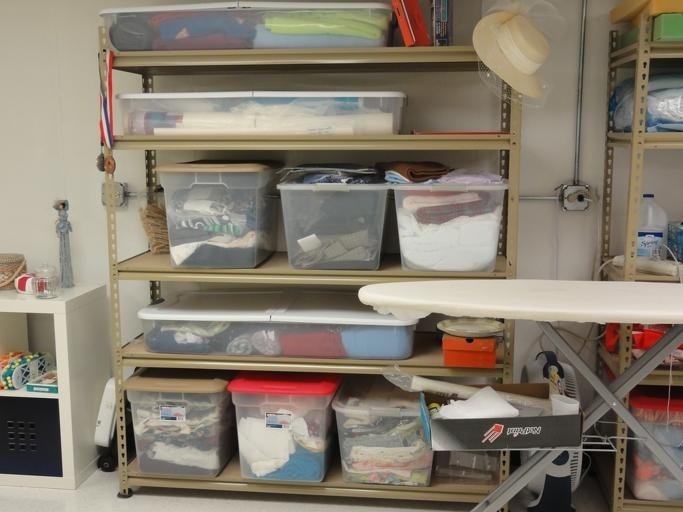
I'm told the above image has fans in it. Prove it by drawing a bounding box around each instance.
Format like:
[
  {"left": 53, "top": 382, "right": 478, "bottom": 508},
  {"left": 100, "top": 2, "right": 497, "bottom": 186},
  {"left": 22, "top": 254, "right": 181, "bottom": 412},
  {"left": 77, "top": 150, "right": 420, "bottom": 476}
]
[{"left": 515, "top": 347, "right": 585, "bottom": 512}]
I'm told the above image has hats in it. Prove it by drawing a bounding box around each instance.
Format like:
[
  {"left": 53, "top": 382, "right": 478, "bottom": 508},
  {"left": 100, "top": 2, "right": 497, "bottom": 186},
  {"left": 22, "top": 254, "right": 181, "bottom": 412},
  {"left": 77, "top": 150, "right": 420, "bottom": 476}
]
[{"left": 472, "top": 12, "right": 549, "bottom": 97}]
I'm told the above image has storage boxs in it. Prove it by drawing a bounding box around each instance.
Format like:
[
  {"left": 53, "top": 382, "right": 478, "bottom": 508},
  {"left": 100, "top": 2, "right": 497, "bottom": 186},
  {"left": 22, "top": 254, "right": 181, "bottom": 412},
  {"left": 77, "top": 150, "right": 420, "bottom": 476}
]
[
  {"left": 226, "top": 371, "right": 341, "bottom": 485},
  {"left": 120, "top": 368, "right": 234, "bottom": 483},
  {"left": 116, "top": 87, "right": 410, "bottom": 144},
  {"left": 330, "top": 379, "right": 437, "bottom": 491},
  {"left": 271, "top": 173, "right": 393, "bottom": 272},
  {"left": 133, "top": 284, "right": 422, "bottom": 359},
  {"left": 150, "top": 158, "right": 280, "bottom": 274},
  {"left": 626, "top": 389, "right": 683, "bottom": 508},
  {"left": 389, "top": 171, "right": 512, "bottom": 276},
  {"left": 95, "top": 0, "right": 401, "bottom": 50}
]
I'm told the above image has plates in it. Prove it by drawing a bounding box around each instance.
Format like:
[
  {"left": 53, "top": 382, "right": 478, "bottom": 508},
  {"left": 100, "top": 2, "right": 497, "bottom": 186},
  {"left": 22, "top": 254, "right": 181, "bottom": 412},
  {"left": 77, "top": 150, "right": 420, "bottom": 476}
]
[{"left": 434, "top": 317, "right": 507, "bottom": 339}]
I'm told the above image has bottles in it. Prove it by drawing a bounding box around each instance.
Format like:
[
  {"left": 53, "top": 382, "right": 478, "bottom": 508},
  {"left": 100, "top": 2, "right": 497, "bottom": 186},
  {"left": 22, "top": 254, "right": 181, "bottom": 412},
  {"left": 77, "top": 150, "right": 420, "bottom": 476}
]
[{"left": 30, "top": 261, "right": 58, "bottom": 300}]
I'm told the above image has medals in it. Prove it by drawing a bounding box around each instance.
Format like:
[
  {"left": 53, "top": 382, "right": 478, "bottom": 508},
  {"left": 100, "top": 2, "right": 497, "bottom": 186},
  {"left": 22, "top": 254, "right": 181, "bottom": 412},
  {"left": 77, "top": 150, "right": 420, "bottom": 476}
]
[
  {"left": 103, "top": 156, "right": 116, "bottom": 174},
  {"left": 96, "top": 153, "right": 105, "bottom": 172}
]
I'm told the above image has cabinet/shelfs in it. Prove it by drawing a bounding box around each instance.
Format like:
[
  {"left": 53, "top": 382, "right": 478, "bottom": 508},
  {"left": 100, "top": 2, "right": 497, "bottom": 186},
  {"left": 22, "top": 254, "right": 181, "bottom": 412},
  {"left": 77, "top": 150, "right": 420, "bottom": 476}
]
[
  {"left": 0, "top": 281, "right": 113, "bottom": 492},
  {"left": 105, "top": 37, "right": 528, "bottom": 510},
  {"left": 594, "top": 1, "right": 683, "bottom": 512}
]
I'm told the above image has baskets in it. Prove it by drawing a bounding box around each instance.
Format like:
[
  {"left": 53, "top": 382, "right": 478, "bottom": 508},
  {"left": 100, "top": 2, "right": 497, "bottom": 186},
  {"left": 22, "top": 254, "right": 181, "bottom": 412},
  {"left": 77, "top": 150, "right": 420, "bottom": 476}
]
[{"left": 0, "top": 254, "right": 26, "bottom": 288}]
